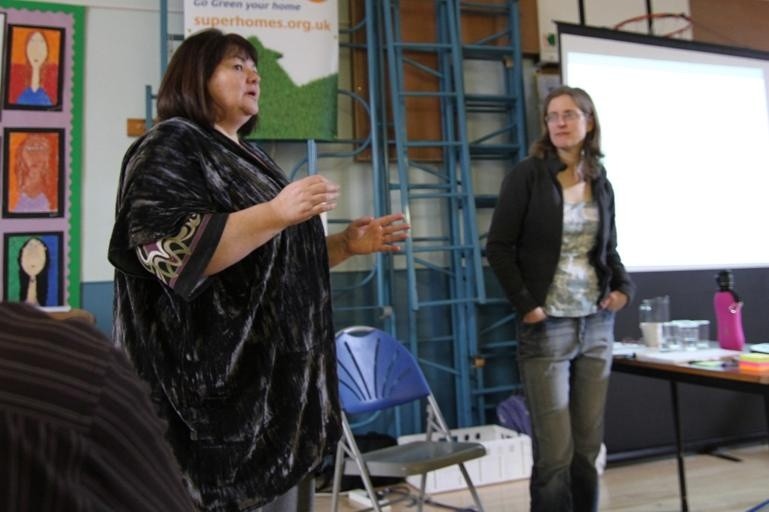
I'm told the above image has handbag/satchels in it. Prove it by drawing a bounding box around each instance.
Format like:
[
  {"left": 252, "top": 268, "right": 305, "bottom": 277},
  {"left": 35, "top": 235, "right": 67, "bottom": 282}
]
[{"left": 334, "top": 431, "right": 397, "bottom": 490}]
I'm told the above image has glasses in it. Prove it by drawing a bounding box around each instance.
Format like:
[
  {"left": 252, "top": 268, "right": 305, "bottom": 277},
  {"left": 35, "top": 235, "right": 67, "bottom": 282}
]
[{"left": 544, "top": 110, "right": 587, "bottom": 124}]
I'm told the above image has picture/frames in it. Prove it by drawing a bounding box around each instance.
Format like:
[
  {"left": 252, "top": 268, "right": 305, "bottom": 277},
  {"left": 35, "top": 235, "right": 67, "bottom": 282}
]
[{"left": 4, "top": 24, "right": 64, "bottom": 307}]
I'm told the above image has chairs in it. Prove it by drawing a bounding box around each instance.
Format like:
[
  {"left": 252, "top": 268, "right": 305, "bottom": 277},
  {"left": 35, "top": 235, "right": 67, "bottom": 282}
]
[{"left": 331, "top": 325, "right": 485, "bottom": 511}]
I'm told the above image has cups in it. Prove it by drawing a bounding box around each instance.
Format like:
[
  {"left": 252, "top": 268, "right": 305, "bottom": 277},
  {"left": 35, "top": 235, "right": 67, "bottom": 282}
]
[{"left": 639, "top": 299, "right": 710, "bottom": 353}]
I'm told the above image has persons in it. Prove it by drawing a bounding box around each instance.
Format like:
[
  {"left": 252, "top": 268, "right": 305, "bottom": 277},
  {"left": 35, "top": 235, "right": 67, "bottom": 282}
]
[
  {"left": 485, "top": 81, "right": 635, "bottom": 512},
  {"left": 106, "top": 24, "right": 411, "bottom": 512}
]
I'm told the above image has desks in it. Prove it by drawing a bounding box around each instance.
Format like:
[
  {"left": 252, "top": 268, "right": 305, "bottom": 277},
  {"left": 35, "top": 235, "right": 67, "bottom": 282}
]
[{"left": 611, "top": 341, "right": 769, "bottom": 512}]
